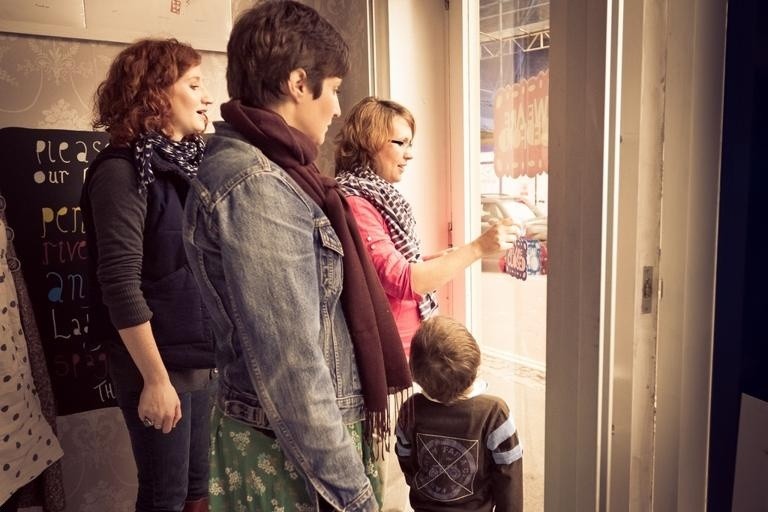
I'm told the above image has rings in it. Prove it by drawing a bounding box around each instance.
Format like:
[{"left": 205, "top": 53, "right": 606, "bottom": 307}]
[{"left": 144, "top": 418, "right": 152, "bottom": 429}]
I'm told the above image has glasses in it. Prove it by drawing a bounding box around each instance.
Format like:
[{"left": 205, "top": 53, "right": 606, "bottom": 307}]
[{"left": 388, "top": 138, "right": 413, "bottom": 148}]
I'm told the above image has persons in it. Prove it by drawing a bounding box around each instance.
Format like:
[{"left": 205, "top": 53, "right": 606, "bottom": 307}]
[
  {"left": 82, "top": 38, "right": 216, "bottom": 512},
  {"left": 392, "top": 316, "right": 523, "bottom": 512},
  {"left": 524, "top": 217, "right": 546, "bottom": 242},
  {"left": 334, "top": 97, "right": 520, "bottom": 366},
  {"left": 180, "top": 1, "right": 413, "bottom": 511}
]
